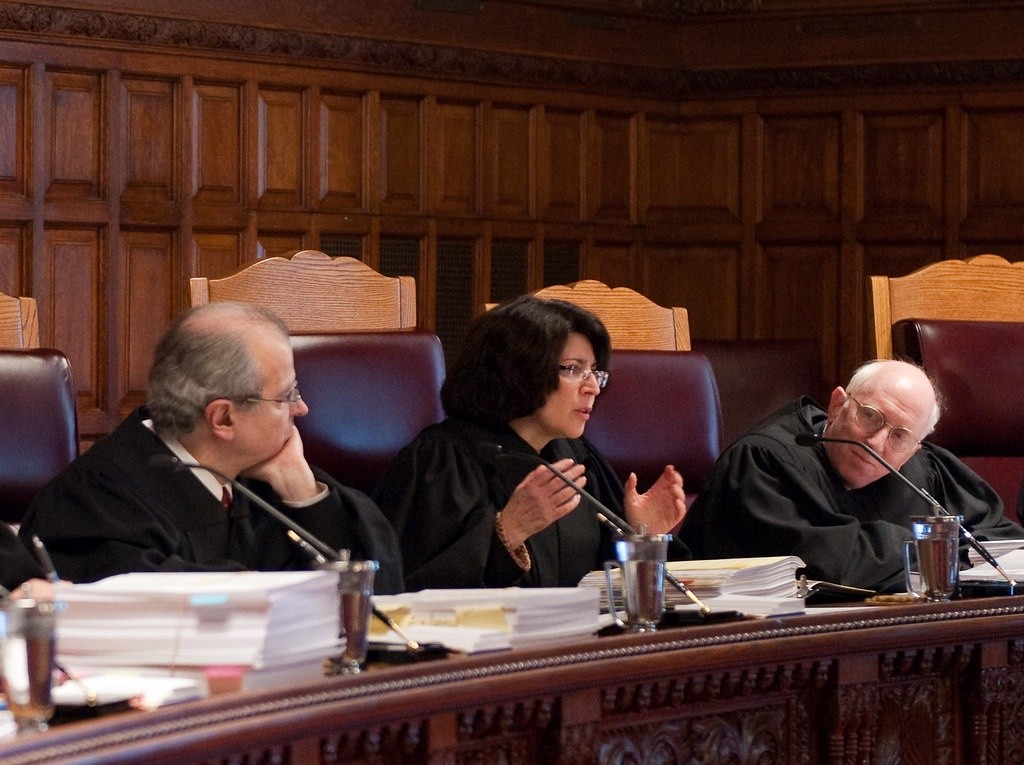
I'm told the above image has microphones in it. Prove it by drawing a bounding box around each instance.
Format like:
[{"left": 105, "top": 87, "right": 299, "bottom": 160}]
[
  {"left": 794, "top": 432, "right": 1024, "bottom": 598},
  {"left": 148, "top": 454, "right": 448, "bottom": 665},
  {"left": 478, "top": 441, "right": 747, "bottom": 636}
]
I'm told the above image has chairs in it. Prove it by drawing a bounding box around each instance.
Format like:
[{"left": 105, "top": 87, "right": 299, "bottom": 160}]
[
  {"left": 863, "top": 255, "right": 1024, "bottom": 529},
  {"left": 190, "top": 249, "right": 448, "bottom": 493},
  {"left": 0, "top": 292, "right": 80, "bottom": 525},
  {"left": 479, "top": 278, "right": 725, "bottom": 536}
]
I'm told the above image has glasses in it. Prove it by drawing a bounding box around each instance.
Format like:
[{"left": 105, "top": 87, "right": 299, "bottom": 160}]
[
  {"left": 847, "top": 392, "right": 922, "bottom": 453},
  {"left": 200, "top": 384, "right": 301, "bottom": 420},
  {"left": 558, "top": 363, "right": 610, "bottom": 388}
]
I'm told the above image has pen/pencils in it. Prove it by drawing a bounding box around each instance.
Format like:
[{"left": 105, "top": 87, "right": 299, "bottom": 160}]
[
  {"left": 287, "top": 526, "right": 420, "bottom": 649},
  {"left": 32, "top": 533, "right": 58, "bottom": 582},
  {"left": 597, "top": 512, "right": 709, "bottom": 612}
]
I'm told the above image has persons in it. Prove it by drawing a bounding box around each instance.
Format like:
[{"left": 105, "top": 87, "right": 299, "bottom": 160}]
[
  {"left": 16, "top": 301, "right": 404, "bottom": 597},
  {"left": 674, "top": 355, "right": 1024, "bottom": 599},
  {"left": 369, "top": 294, "right": 687, "bottom": 591},
  {"left": 0, "top": 520, "right": 75, "bottom": 603}
]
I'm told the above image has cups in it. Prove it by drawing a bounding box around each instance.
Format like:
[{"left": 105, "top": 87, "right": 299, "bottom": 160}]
[
  {"left": 0, "top": 587, "right": 67, "bottom": 740},
  {"left": 313, "top": 550, "right": 379, "bottom": 675},
  {"left": 606, "top": 526, "right": 673, "bottom": 635},
  {"left": 903, "top": 507, "right": 964, "bottom": 600}
]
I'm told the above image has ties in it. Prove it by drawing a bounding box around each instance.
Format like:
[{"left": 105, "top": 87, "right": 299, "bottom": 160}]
[{"left": 219, "top": 486, "right": 231, "bottom": 512}]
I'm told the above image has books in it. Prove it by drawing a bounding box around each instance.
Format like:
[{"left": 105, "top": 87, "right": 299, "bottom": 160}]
[
  {"left": 958, "top": 538, "right": 1024, "bottom": 583},
  {"left": 51, "top": 568, "right": 345, "bottom": 702},
  {"left": 576, "top": 554, "right": 807, "bottom": 609},
  {"left": 366, "top": 583, "right": 630, "bottom": 654}
]
